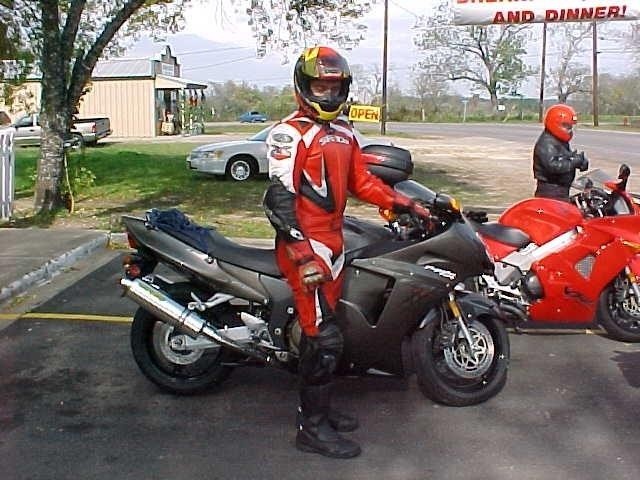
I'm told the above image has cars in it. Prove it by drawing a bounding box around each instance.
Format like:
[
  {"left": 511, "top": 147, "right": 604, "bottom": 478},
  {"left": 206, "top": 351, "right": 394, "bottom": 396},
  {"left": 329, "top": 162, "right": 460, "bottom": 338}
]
[
  {"left": 188, "top": 120, "right": 394, "bottom": 182},
  {"left": 237, "top": 110, "right": 268, "bottom": 124}
]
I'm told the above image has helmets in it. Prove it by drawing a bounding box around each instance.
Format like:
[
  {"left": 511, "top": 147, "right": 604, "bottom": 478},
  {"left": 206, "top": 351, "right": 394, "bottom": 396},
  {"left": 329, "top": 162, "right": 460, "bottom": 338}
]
[
  {"left": 294, "top": 48, "right": 353, "bottom": 121},
  {"left": 544, "top": 105, "right": 577, "bottom": 142}
]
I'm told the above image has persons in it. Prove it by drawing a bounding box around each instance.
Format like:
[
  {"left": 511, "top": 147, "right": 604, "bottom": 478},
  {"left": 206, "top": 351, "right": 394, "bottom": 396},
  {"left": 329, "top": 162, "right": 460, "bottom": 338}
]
[
  {"left": 533, "top": 104, "right": 589, "bottom": 200},
  {"left": 262, "top": 44, "right": 434, "bottom": 459}
]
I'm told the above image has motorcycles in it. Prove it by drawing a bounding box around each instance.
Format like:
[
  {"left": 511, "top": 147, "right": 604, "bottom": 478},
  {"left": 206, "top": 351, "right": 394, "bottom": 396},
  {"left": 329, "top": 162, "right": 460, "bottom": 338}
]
[
  {"left": 118, "top": 190, "right": 509, "bottom": 406},
  {"left": 365, "top": 144, "right": 639, "bottom": 342}
]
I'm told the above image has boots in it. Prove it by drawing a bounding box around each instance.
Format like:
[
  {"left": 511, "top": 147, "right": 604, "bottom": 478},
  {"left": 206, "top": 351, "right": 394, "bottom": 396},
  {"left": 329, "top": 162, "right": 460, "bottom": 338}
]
[{"left": 296, "top": 385, "right": 361, "bottom": 459}]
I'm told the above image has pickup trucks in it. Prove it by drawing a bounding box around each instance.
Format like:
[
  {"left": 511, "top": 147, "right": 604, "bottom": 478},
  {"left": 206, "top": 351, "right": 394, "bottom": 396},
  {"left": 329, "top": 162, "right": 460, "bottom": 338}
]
[{"left": 9, "top": 110, "right": 115, "bottom": 151}]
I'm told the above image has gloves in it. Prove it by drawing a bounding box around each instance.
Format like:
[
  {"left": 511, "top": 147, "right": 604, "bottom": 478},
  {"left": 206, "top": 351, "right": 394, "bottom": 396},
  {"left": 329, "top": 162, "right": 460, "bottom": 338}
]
[
  {"left": 567, "top": 150, "right": 588, "bottom": 171},
  {"left": 296, "top": 253, "right": 324, "bottom": 292}
]
